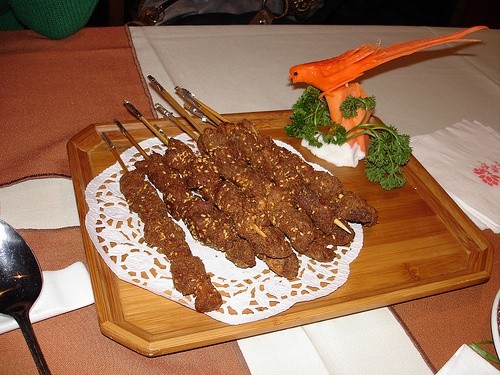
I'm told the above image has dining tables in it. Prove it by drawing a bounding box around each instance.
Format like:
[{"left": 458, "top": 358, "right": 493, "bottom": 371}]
[{"left": 0, "top": 25, "right": 500, "bottom": 375}]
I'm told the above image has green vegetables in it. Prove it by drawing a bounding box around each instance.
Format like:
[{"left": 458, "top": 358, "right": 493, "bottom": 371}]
[{"left": 284, "top": 85, "right": 414, "bottom": 191}]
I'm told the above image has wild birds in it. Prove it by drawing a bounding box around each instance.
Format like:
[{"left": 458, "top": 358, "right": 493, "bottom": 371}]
[{"left": 289, "top": 26, "right": 488, "bottom": 99}]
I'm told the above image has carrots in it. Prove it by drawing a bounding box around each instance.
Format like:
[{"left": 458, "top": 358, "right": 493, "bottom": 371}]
[{"left": 288, "top": 25, "right": 489, "bottom": 158}]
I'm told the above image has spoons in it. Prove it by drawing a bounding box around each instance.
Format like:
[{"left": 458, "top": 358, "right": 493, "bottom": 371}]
[{"left": 0, "top": 219, "right": 52, "bottom": 375}]
